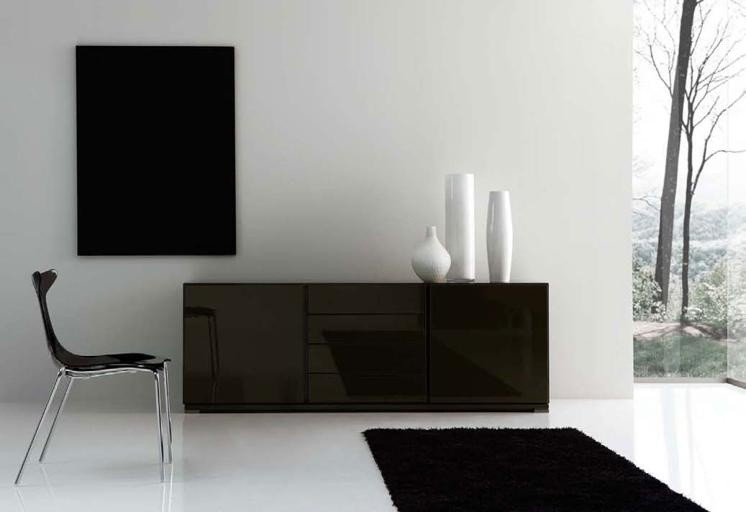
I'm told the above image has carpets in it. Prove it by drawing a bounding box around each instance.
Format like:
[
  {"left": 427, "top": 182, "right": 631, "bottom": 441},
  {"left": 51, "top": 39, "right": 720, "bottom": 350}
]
[{"left": 359, "top": 427, "right": 711, "bottom": 512}]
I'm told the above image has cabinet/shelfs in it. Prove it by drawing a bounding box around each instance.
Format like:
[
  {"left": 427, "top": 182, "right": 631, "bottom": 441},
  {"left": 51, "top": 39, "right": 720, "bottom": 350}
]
[{"left": 176, "top": 281, "right": 550, "bottom": 411}]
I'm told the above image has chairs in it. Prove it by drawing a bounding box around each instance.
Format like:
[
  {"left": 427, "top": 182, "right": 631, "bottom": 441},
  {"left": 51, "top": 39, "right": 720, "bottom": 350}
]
[{"left": 15, "top": 266, "right": 174, "bottom": 484}]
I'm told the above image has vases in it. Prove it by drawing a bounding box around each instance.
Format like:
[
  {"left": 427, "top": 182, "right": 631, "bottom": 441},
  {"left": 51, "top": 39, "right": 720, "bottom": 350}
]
[{"left": 412, "top": 169, "right": 516, "bottom": 284}]
